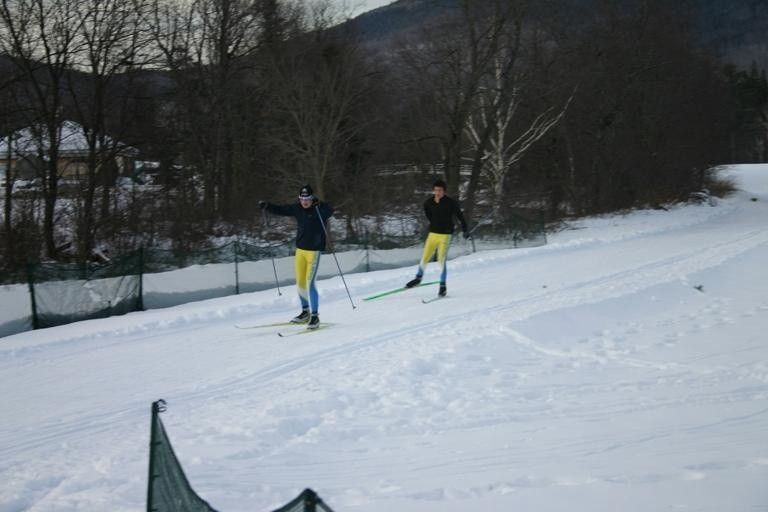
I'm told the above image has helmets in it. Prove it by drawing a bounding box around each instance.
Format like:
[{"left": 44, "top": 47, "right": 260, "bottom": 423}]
[{"left": 299, "top": 185, "right": 313, "bottom": 198}]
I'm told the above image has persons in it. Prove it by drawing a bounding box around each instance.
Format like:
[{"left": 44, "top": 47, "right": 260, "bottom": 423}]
[
  {"left": 407, "top": 181, "right": 470, "bottom": 295},
  {"left": 258, "top": 185, "right": 333, "bottom": 329}
]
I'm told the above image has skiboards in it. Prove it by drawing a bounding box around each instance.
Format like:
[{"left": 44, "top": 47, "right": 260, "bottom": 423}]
[
  {"left": 361, "top": 281, "right": 439, "bottom": 304},
  {"left": 234, "top": 323, "right": 337, "bottom": 337}
]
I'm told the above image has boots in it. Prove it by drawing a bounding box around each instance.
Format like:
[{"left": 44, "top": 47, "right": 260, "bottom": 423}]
[
  {"left": 439, "top": 283, "right": 446, "bottom": 296},
  {"left": 406, "top": 275, "right": 422, "bottom": 287},
  {"left": 307, "top": 311, "right": 319, "bottom": 329},
  {"left": 292, "top": 305, "right": 310, "bottom": 324}
]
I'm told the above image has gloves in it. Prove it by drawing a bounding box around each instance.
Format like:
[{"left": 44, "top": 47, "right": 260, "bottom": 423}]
[
  {"left": 463, "top": 230, "right": 469, "bottom": 238},
  {"left": 311, "top": 197, "right": 319, "bottom": 208},
  {"left": 257, "top": 201, "right": 266, "bottom": 208}
]
